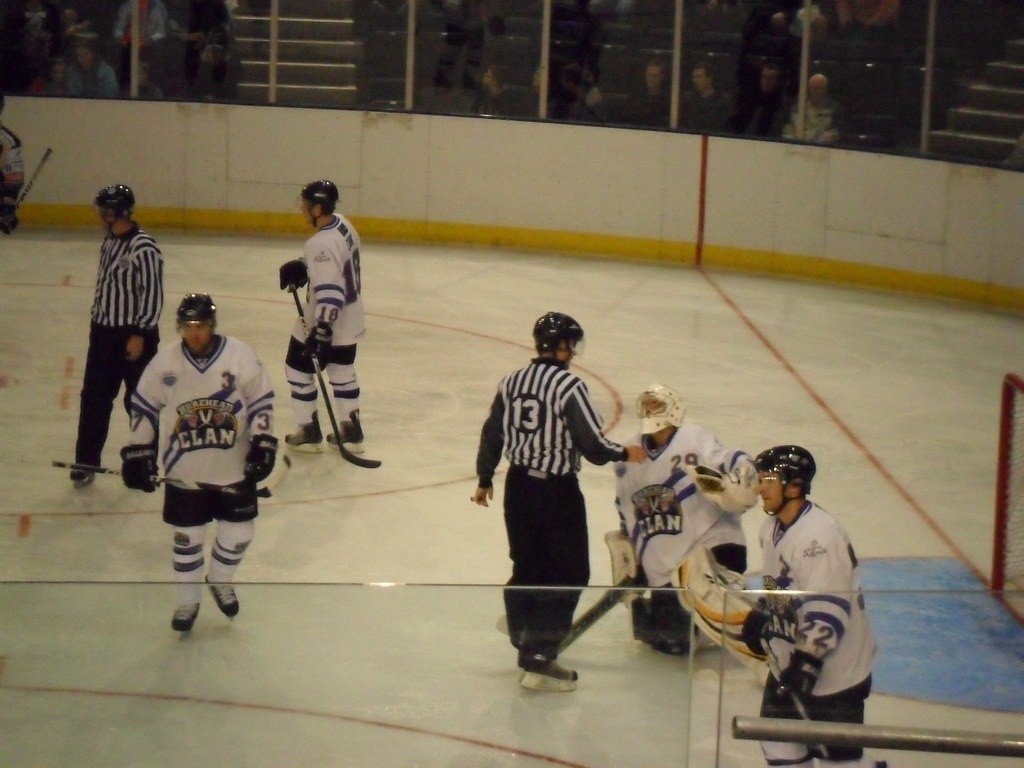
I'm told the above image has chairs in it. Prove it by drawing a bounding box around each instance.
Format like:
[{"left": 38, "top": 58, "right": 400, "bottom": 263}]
[{"left": 355, "top": 1, "right": 960, "bottom": 144}]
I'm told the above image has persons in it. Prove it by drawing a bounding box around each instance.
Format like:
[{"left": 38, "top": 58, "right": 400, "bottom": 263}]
[
  {"left": 745, "top": 445, "right": 878, "bottom": 768},
  {"left": 118, "top": 293, "right": 278, "bottom": 641},
  {"left": 0, "top": 0, "right": 252, "bottom": 105},
  {"left": 612, "top": 385, "right": 763, "bottom": 656},
  {"left": 473, "top": 312, "right": 645, "bottom": 691},
  {"left": 277, "top": 179, "right": 368, "bottom": 456},
  {"left": 0, "top": 95, "right": 24, "bottom": 234},
  {"left": 355, "top": 0, "right": 973, "bottom": 161},
  {"left": 69, "top": 185, "right": 164, "bottom": 488}
]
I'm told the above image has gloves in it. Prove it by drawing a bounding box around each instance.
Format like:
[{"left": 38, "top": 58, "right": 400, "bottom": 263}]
[
  {"left": 244, "top": 433, "right": 278, "bottom": 482},
  {"left": 119, "top": 444, "right": 160, "bottom": 492},
  {"left": 741, "top": 597, "right": 774, "bottom": 662},
  {"left": 301, "top": 322, "right": 332, "bottom": 370},
  {"left": 777, "top": 648, "right": 823, "bottom": 714},
  {"left": 1, "top": 204, "right": 18, "bottom": 235},
  {"left": 279, "top": 260, "right": 308, "bottom": 293}
]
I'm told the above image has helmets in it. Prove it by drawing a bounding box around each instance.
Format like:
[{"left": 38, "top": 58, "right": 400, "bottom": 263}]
[
  {"left": 300, "top": 179, "right": 338, "bottom": 205},
  {"left": 532, "top": 312, "right": 583, "bottom": 341},
  {"left": 176, "top": 293, "right": 216, "bottom": 327},
  {"left": 94, "top": 184, "right": 134, "bottom": 209},
  {"left": 755, "top": 445, "right": 817, "bottom": 495},
  {"left": 634, "top": 382, "right": 686, "bottom": 435}
]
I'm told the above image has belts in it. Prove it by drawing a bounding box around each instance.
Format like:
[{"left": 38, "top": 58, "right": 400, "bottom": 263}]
[{"left": 527, "top": 468, "right": 547, "bottom": 478}]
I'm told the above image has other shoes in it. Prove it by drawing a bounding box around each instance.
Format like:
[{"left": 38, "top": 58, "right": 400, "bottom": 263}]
[{"left": 69, "top": 469, "right": 95, "bottom": 488}]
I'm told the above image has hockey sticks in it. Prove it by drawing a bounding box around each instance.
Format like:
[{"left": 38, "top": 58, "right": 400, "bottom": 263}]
[
  {"left": 50, "top": 454, "right": 291, "bottom": 498},
  {"left": 495, "top": 577, "right": 636, "bottom": 657},
  {"left": 290, "top": 282, "right": 382, "bottom": 469},
  {"left": 16, "top": 148, "right": 52, "bottom": 209},
  {"left": 760, "top": 637, "right": 830, "bottom": 760}
]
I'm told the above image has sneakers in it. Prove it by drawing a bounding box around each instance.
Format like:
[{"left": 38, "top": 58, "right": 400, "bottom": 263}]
[
  {"left": 327, "top": 409, "right": 365, "bottom": 453},
  {"left": 205, "top": 574, "right": 239, "bottom": 622},
  {"left": 518, "top": 650, "right": 578, "bottom": 691},
  {"left": 284, "top": 410, "right": 324, "bottom": 453},
  {"left": 171, "top": 603, "right": 200, "bottom": 639}
]
